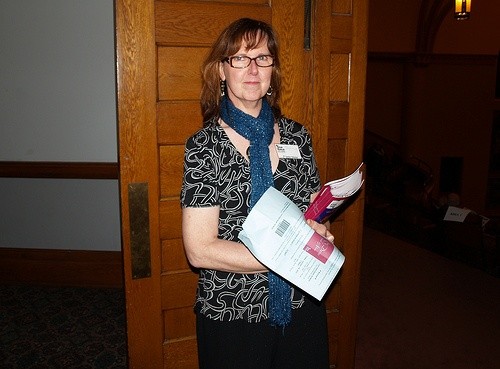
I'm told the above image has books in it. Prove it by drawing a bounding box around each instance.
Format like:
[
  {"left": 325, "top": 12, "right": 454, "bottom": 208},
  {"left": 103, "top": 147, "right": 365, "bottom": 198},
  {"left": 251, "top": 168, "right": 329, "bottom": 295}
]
[{"left": 303, "top": 162, "right": 365, "bottom": 224}]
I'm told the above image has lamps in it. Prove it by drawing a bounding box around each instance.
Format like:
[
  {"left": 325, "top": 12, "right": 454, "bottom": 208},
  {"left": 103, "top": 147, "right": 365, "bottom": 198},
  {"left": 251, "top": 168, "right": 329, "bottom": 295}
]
[{"left": 454, "top": 0, "right": 472, "bottom": 20}]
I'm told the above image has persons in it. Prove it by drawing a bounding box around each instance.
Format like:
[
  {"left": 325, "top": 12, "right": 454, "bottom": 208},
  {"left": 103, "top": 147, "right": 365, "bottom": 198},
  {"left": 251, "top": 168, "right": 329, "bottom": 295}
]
[{"left": 180, "top": 17, "right": 335, "bottom": 369}]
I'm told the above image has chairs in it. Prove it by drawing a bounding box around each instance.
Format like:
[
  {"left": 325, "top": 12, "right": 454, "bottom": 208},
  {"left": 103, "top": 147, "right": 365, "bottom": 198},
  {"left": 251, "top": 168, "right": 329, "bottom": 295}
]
[{"left": 365, "top": 193, "right": 497, "bottom": 273}]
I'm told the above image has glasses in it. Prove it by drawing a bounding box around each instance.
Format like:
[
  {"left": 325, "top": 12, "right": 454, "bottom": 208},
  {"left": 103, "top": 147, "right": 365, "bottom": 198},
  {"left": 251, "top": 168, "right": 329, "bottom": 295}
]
[{"left": 220, "top": 55, "right": 274, "bottom": 69}]
